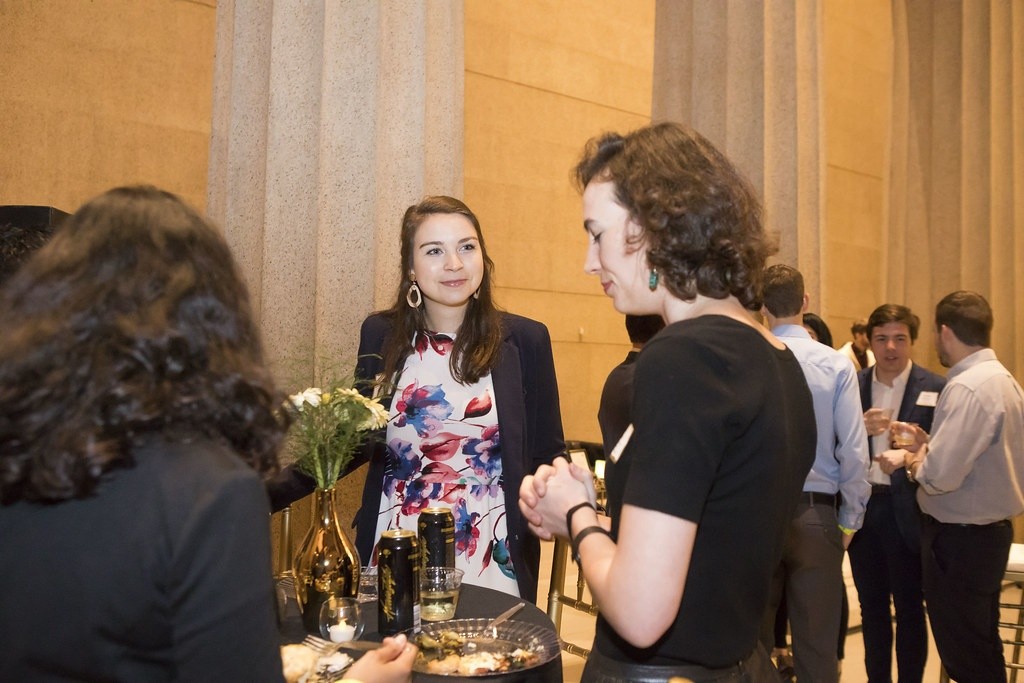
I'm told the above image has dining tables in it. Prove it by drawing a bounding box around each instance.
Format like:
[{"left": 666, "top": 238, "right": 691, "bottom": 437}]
[{"left": 273, "top": 577, "right": 566, "bottom": 683}]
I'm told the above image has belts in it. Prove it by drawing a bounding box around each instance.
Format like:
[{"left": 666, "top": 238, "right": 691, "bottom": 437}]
[
  {"left": 802, "top": 491, "right": 840, "bottom": 511},
  {"left": 922, "top": 513, "right": 1011, "bottom": 528}
]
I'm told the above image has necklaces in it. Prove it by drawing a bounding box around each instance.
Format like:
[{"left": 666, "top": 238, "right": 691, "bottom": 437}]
[{"left": 422, "top": 308, "right": 462, "bottom": 351}]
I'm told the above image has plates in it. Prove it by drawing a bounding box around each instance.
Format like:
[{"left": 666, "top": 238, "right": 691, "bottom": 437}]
[
  {"left": 393, "top": 617, "right": 564, "bottom": 677},
  {"left": 277, "top": 566, "right": 378, "bottom": 603}
]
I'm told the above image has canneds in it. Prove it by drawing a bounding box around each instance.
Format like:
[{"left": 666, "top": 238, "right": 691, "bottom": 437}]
[
  {"left": 377, "top": 529, "right": 423, "bottom": 639},
  {"left": 417, "top": 507, "right": 458, "bottom": 591}
]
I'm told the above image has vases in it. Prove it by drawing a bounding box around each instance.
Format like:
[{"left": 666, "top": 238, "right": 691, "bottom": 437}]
[{"left": 291, "top": 489, "right": 360, "bottom": 637}]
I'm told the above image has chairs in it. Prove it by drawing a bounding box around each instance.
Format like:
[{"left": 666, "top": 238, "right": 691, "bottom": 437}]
[{"left": 545, "top": 440, "right": 612, "bottom": 662}]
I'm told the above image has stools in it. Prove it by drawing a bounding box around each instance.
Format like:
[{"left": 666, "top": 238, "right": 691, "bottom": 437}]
[{"left": 939, "top": 542, "right": 1024, "bottom": 683}]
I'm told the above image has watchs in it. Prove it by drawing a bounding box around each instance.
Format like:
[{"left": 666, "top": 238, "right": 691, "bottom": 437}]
[{"left": 906, "top": 460, "right": 918, "bottom": 482}]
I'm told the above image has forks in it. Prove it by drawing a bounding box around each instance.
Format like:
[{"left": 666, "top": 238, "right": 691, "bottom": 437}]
[
  {"left": 466, "top": 602, "right": 526, "bottom": 645},
  {"left": 300, "top": 635, "right": 383, "bottom": 656}
]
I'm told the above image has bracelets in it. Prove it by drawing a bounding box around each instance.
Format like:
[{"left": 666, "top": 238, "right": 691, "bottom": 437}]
[
  {"left": 566, "top": 502, "right": 596, "bottom": 539},
  {"left": 838, "top": 524, "right": 857, "bottom": 535},
  {"left": 572, "top": 526, "right": 615, "bottom": 569}
]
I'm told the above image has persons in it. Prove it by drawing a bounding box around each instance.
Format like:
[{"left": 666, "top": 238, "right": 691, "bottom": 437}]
[
  {"left": 597, "top": 264, "right": 1024, "bottom": 683},
  {"left": 0, "top": 183, "right": 291, "bottom": 683},
  {"left": 264, "top": 196, "right": 572, "bottom": 606},
  {"left": 519, "top": 120, "right": 818, "bottom": 683}
]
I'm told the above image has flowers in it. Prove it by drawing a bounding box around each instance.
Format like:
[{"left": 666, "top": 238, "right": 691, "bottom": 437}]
[{"left": 270, "top": 351, "right": 407, "bottom": 489}]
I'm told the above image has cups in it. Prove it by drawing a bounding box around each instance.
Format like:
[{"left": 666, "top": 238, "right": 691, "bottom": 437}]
[
  {"left": 319, "top": 597, "right": 365, "bottom": 642},
  {"left": 894, "top": 422, "right": 919, "bottom": 446},
  {"left": 870, "top": 408, "right": 893, "bottom": 432},
  {"left": 421, "top": 566, "right": 465, "bottom": 621}
]
